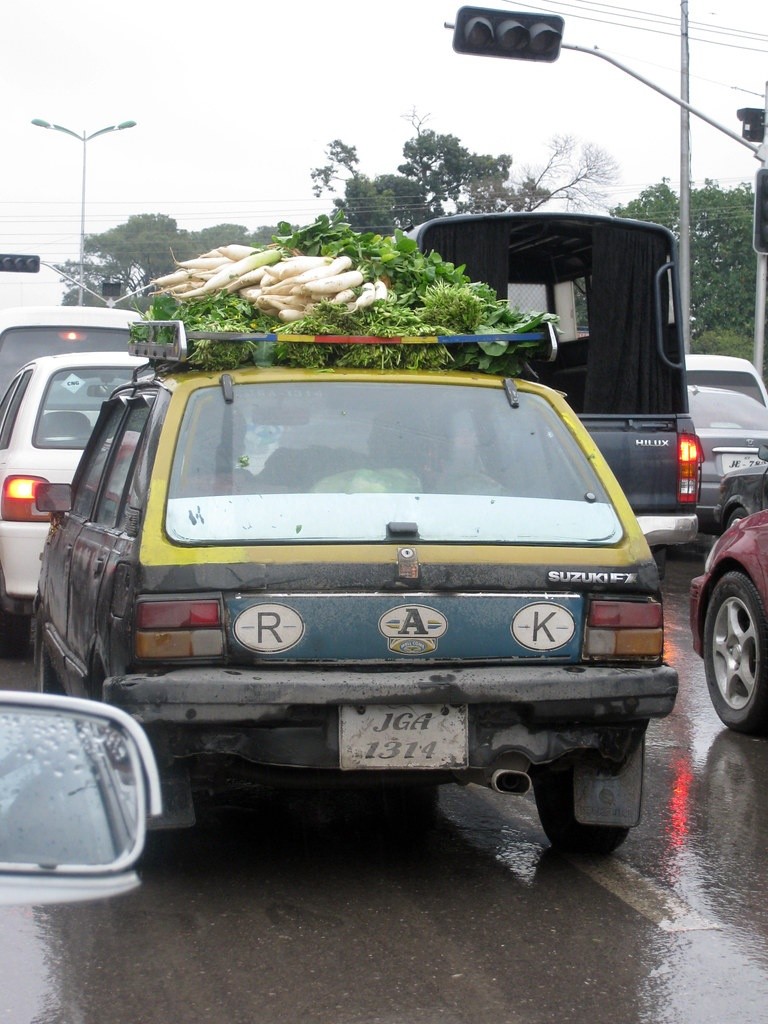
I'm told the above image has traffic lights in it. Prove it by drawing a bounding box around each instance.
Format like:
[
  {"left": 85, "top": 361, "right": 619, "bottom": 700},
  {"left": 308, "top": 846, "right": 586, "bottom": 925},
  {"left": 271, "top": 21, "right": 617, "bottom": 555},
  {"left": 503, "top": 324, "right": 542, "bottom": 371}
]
[
  {"left": 0, "top": 254, "right": 40, "bottom": 273},
  {"left": 752, "top": 168, "right": 768, "bottom": 255},
  {"left": 453, "top": 5, "right": 566, "bottom": 62}
]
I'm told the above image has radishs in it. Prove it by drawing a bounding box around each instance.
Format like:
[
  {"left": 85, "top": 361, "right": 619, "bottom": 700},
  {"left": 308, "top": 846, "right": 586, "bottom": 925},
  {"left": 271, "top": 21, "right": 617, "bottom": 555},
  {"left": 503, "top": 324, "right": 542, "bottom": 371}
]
[{"left": 150, "top": 245, "right": 390, "bottom": 324}]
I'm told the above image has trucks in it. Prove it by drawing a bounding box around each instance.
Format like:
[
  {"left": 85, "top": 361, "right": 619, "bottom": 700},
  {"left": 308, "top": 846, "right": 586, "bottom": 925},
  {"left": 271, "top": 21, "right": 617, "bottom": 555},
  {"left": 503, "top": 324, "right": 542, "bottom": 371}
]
[{"left": 383, "top": 211, "right": 704, "bottom": 583}]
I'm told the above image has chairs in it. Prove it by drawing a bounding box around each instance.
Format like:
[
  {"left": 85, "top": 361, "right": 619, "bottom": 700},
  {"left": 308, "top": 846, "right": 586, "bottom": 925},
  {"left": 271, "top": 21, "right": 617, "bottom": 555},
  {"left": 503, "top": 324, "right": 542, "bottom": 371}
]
[{"left": 41, "top": 409, "right": 94, "bottom": 444}]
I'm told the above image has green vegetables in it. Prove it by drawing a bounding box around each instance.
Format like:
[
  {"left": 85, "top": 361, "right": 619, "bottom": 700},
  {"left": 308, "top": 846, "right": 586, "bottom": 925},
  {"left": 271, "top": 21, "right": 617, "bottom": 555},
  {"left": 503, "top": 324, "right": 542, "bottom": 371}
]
[{"left": 124, "top": 281, "right": 560, "bottom": 377}]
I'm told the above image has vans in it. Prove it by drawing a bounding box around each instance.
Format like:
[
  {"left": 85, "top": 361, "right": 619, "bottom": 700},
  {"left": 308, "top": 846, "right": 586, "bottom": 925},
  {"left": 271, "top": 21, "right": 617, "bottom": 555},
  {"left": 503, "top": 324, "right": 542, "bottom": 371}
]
[{"left": 1, "top": 305, "right": 147, "bottom": 400}]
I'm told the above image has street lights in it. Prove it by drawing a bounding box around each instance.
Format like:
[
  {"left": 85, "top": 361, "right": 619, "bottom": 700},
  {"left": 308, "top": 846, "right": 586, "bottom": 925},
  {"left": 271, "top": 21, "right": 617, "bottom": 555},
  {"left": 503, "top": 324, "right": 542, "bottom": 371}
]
[{"left": 31, "top": 118, "right": 136, "bottom": 306}]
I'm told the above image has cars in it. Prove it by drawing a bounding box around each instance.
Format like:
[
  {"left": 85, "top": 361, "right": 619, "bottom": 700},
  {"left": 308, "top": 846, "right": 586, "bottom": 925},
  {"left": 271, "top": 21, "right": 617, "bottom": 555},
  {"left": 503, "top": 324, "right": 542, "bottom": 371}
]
[{"left": 690, "top": 445, "right": 768, "bottom": 734}]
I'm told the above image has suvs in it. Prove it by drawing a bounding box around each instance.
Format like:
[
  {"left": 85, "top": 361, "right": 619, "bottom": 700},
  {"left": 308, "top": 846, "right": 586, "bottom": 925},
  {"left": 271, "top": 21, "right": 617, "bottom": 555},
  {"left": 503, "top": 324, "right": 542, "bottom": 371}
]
[
  {"left": 0, "top": 353, "right": 156, "bottom": 660},
  {"left": 686, "top": 354, "right": 768, "bottom": 522},
  {"left": 32, "top": 322, "right": 678, "bottom": 860}
]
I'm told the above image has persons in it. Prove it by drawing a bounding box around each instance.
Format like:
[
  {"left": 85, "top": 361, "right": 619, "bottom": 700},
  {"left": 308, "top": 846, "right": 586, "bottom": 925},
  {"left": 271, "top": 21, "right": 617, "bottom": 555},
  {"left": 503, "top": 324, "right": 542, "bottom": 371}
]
[{"left": 188, "top": 407, "right": 247, "bottom": 477}]
